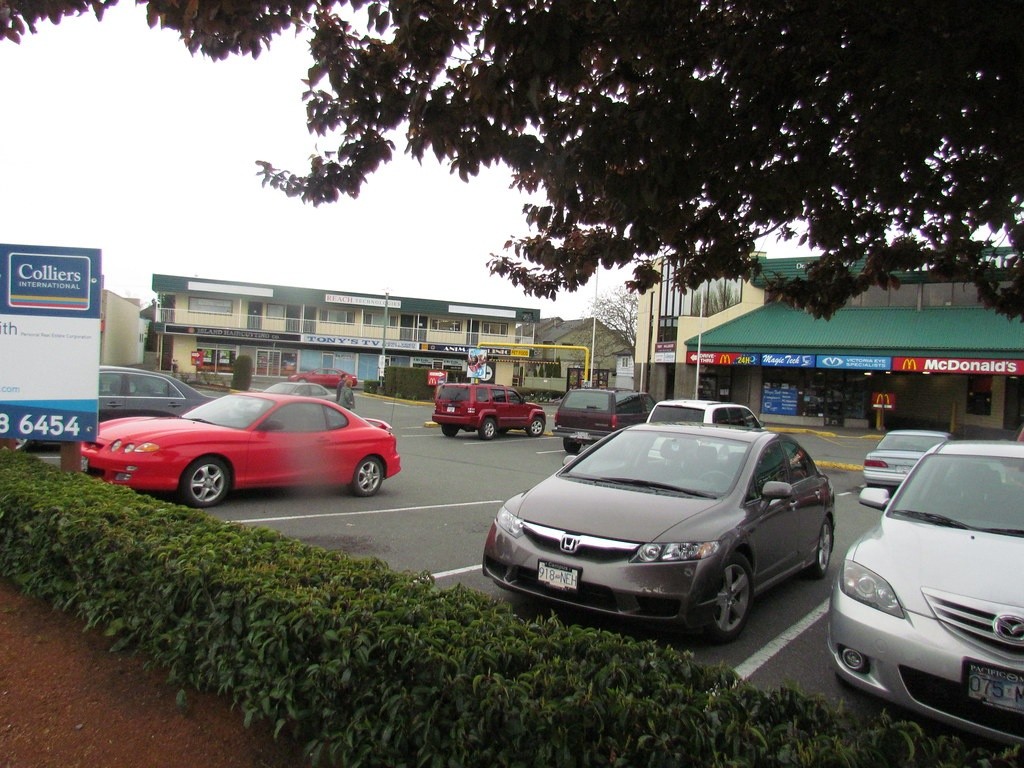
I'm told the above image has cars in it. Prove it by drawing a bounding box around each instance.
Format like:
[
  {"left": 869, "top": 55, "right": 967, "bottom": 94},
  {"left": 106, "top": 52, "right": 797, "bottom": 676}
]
[
  {"left": 261, "top": 381, "right": 337, "bottom": 403},
  {"left": 861, "top": 428, "right": 954, "bottom": 498},
  {"left": 81, "top": 391, "right": 402, "bottom": 509},
  {"left": 288, "top": 367, "right": 358, "bottom": 388},
  {"left": 480, "top": 420, "right": 837, "bottom": 646},
  {"left": 824, "top": 439, "right": 1024, "bottom": 755}
]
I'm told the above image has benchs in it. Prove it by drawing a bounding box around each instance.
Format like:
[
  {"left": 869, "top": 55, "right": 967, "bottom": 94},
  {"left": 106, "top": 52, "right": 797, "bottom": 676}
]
[
  {"left": 134, "top": 385, "right": 167, "bottom": 395},
  {"left": 686, "top": 447, "right": 742, "bottom": 480}
]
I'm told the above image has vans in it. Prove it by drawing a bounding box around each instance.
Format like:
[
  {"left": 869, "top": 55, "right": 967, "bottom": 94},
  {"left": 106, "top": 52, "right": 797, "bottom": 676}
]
[{"left": 642, "top": 398, "right": 768, "bottom": 460}]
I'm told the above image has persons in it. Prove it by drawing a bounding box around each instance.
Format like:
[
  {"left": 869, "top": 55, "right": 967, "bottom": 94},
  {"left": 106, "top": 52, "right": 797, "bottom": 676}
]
[
  {"left": 339, "top": 380, "right": 355, "bottom": 410},
  {"left": 336, "top": 374, "right": 349, "bottom": 404}
]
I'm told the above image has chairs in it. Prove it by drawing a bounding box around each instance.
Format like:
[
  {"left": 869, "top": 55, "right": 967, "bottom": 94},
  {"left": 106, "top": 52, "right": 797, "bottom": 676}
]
[
  {"left": 720, "top": 453, "right": 745, "bottom": 480},
  {"left": 108, "top": 380, "right": 118, "bottom": 395},
  {"left": 948, "top": 464, "right": 1007, "bottom": 520},
  {"left": 645, "top": 439, "right": 684, "bottom": 478}
]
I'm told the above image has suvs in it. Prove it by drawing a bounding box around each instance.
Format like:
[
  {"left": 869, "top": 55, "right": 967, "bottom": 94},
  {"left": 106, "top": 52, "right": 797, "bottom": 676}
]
[
  {"left": 431, "top": 382, "right": 548, "bottom": 442},
  {"left": 551, "top": 387, "right": 658, "bottom": 455},
  {"left": 15, "top": 364, "right": 221, "bottom": 455}
]
